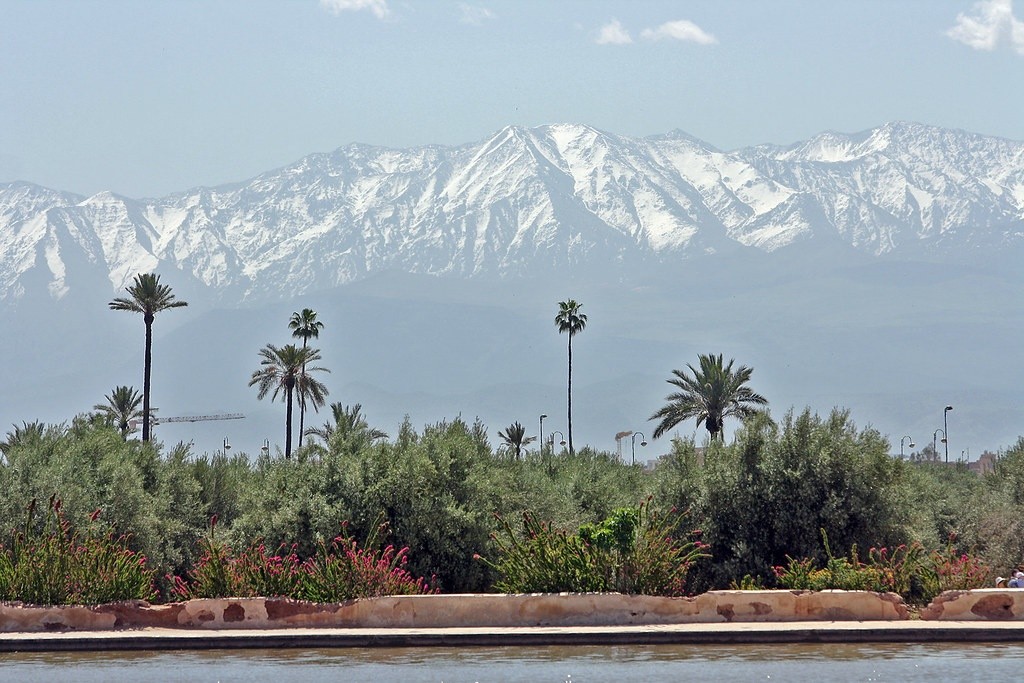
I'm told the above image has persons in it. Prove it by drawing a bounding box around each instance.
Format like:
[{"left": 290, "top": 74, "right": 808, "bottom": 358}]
[
  {"left": 995, "top": 577, "right": 1006, "bottom": 588},
  {"left": 1017, "top": 566, "right": 1024, "bottom": 573},
  {"left": 1016, "top": 572, "right": 1024, "bottom": 588},
  {"left": 1007, "top": 568, "right": 1019, "bottom": 588}
]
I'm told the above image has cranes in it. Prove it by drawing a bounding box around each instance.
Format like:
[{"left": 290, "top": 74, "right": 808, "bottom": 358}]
[{"left": 129, "top": 413, "right": 246, "bottom": 440}]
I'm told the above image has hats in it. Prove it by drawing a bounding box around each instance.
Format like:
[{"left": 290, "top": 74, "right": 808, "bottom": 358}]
[
  {"left": 995, "top": 577, "right": 1004, "bottom": 584},
  {"left": 1017, "top": 572, "right": 1024, "bottom": 578}
]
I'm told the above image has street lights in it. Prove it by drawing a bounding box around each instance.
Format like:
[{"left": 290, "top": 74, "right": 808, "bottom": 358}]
[
  {"left": 224, "top": 436, "right": 231, "bottom": 455},
  {"left": 262, "top": 438, "right": 269, "bottom": 456},
  {"left": 553, "top": 431, "right": 566, "bottom": 453},
  {"left": 540, "top": 414, "right": 547, "bottom": 449},
  {"left": 901, "top": 435, "right": 915, "bottom": 455},
  {"left": 632, "top": 432, "right": 647, "bottom": 463},
  {"left": 944, "top": 406, "right": 953, "bottom": 463},
  {"left": 933, "top": 429, "right": 946, "bottom": 461}
]
